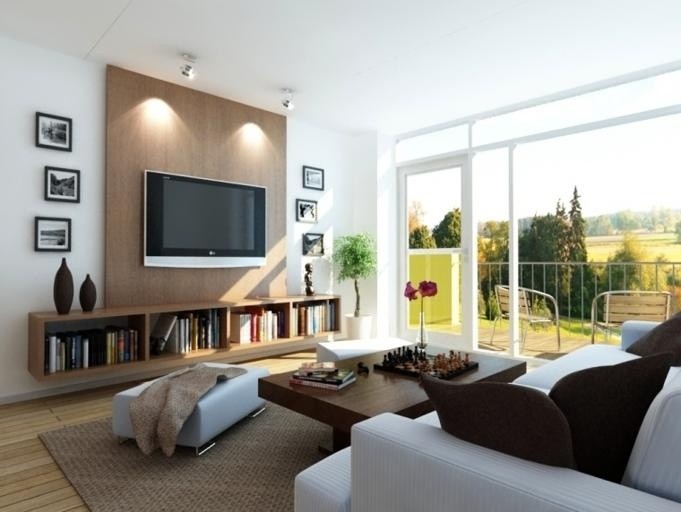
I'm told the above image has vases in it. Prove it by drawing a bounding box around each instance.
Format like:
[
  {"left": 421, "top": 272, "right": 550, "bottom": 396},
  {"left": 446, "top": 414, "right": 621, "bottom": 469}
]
[
  {"left": 415, "top": 313, "right": 429, "bottom": 349},
  {"left": 78, "top": 273, "right": 98, "bottom": 312},
  {"left": 53, "top": 256, "right": 74, "bottom": 315}
]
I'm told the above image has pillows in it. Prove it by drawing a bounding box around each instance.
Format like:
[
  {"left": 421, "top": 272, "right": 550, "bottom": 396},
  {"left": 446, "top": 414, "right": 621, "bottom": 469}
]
[
  {"left": 421, "top": 375, "right": 574, "bottom": 468},
  {"left": 547, "top": 354, "right": 672, "bottom": 483},
  {"left": 625, "top": 313, "right": 681, "bottom": 368}
]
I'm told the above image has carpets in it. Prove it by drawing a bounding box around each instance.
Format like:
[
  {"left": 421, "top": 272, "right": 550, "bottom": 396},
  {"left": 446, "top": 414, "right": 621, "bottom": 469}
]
[{"left": 38, "top": 402, "right": 333, "bottom": 512}]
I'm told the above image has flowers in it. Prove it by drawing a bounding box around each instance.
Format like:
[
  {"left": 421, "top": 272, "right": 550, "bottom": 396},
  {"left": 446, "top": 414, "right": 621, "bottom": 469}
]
[{"left": 404, "top": 279, "right": 437, "bottom": 312}]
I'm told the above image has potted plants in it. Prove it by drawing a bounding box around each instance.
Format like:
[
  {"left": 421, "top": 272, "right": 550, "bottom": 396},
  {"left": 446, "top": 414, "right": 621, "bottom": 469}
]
[{"left": 331, "top": 231, "right": 377, "bottom": 340}]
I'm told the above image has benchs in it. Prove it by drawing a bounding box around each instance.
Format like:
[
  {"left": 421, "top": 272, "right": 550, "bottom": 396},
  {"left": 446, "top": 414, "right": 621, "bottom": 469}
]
[
  {"left": 113, "top": 364, "right": 270, "bottom": 456},
  {"left": 317, "top": 337, "right": 415, "bottom": 363},
  {"left": 591, "top": 290, "right": 672, "bottom": 345}
]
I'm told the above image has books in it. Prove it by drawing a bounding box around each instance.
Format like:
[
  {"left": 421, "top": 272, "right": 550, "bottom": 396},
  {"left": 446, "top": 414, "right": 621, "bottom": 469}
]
[
  {"left": 231, "top": 306, "right": 288, "bottom": 344},
  {"left": 44, "top": 323, "right": 139, "bottom": 376},
  {"left": 290, "top": 300, "right": 339, "bottom": 339},
  {"left": 150, "top": 308, "right": 222, "bottom": 355},
  {"left": 288, "top": 359, "right": 356, "bottom": 394}
]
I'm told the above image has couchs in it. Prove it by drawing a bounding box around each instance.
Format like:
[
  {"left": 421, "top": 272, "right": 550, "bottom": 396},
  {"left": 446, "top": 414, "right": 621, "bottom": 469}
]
[{"left": 294, "top": 320, "right": 680, "bottom": 511}]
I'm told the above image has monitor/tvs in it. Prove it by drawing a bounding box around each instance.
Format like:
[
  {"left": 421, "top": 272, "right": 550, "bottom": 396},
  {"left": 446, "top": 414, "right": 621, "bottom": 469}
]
[{"left": 143, "top": 167, "right": 266, "bottom": 270}]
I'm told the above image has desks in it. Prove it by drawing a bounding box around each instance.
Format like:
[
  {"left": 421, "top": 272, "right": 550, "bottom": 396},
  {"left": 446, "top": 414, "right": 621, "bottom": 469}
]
[
  {"left": 259, "top": 343, "right": 526, "bottom": 456},
  {"left": 26, "top": 294, "right": 343, "bottom": 385}
]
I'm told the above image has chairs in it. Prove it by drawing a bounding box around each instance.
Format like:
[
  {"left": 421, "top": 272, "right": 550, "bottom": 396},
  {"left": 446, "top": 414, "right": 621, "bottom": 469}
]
[{"left": 490, "top": 285, "right": 560, "bottom": 350}]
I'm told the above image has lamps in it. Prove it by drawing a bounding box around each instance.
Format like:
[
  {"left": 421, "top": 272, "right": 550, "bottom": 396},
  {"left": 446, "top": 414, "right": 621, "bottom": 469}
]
[
  {"left": 180, "top": 55, "right": 197, "bottom": 79},
  {"left": 282, "top": 89, "right": 294, "bottom": 110}
]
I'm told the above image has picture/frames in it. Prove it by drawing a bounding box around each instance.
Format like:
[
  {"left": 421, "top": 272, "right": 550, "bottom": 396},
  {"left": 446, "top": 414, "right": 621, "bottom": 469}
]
[
  {"left": 44, "top": 166, "right": 80, "bottom": 203},
  {"left": 34, "top": 216, "right": 71, "bottom": 252},
  {"left": 296, "top": 198, "right": 317, "bottom": 223},
  {"left": 302, "top": 165, "right": 324, "bottom": 191},
  {"left": 302, "top": 233, "right": 324, "bottom": 256},
  {"left": 35, "top": 111, "right": 72, "bottom": 153}
]
